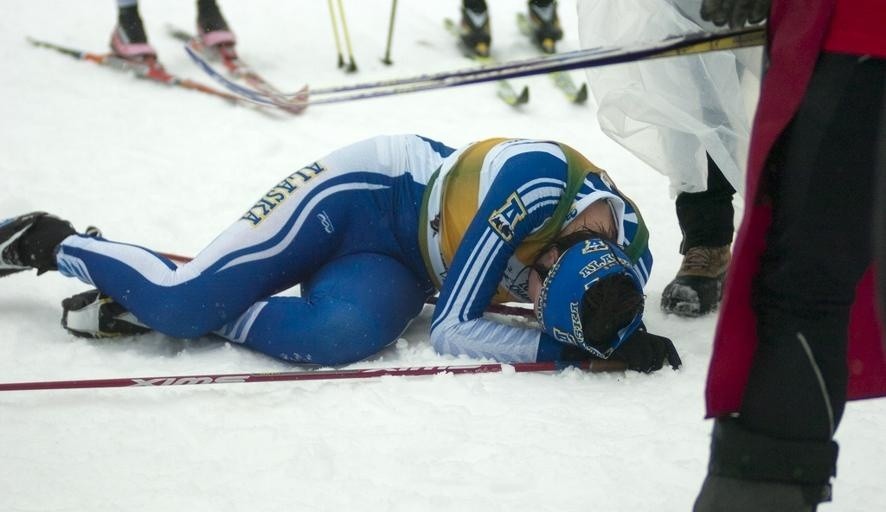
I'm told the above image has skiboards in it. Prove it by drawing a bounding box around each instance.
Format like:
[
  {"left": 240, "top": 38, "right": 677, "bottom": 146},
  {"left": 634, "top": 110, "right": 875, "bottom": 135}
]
[
  {"left": 25, "top": 24, "right": 310, "bottom": 115},
  {"left": 444, "top": 13, "right": 586, "bottom": 105}
]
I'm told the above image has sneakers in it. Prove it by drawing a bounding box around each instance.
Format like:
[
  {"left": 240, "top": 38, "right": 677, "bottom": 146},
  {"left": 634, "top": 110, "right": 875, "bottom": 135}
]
[
  {"left": 112, "top": 25, "right": 156, "bottom": 58},
  {"left": 197, "top": 16, "right": 236, "bottom": 47},
  {"left": 62, "top": 290, "right": 151, "bottom": 338},
  {"left": 529, "top": 3, "right": 561, "bottom": 39},
  {"left": 461, "top": 6, "right": 489, "bottom": 55},
  {"left": 661, "top": 245, "right": 731, "bottom": 316},
  {"left": 1, "top": 211, "right": 50, "bottom": 276}
]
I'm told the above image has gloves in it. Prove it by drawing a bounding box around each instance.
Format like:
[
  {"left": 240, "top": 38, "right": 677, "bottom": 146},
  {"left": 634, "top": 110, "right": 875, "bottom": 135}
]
[
  {"left": 564, "top": 329, "right": 681, "bottom": 373},
  {"left": 700, "top": 0, "right": 770, "bottom": 29}
]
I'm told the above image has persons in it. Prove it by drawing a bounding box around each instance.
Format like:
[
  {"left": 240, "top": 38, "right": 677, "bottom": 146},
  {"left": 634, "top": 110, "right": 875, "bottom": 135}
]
[
  {"left": 663, "top": 1, "right": 743, "bottom": 319},
  {"left": 111, "top": 0, "right": 236, "bottom": 59},
  {"left": 694, "top": 0, "right": 885, "bottom": 512},
  {"left": 459, "top": 0, "right": 564, "bottom": 58},
  {"left": 0, "top": 132, "right": 681, "bottom": 373}
]
